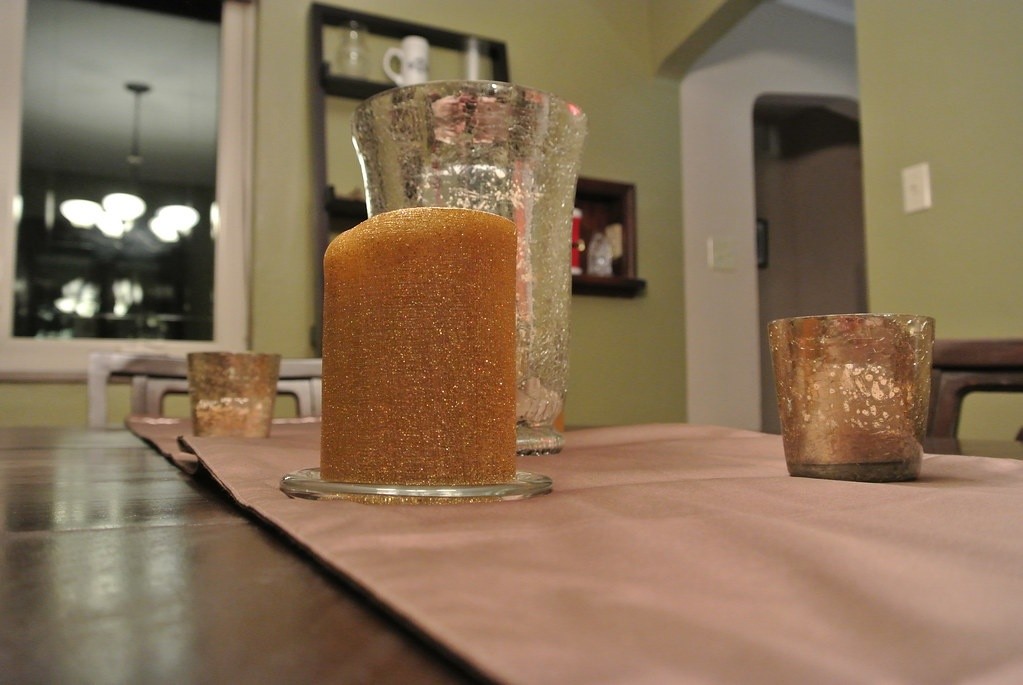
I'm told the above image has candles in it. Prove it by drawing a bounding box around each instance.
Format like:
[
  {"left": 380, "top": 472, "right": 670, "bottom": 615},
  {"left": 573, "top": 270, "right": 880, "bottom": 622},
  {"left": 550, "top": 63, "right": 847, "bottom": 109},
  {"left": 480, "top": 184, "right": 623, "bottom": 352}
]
[{"left": 323, "top": 205, "right": 519, "bottom": 482}]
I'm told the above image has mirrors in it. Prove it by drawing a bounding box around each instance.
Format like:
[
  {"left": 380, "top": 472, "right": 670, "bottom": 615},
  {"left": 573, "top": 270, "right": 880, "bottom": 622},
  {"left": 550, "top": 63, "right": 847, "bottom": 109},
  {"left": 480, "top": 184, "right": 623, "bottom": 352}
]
[{"left": 0, "top": 0, "right": 261, "bottom": 388}]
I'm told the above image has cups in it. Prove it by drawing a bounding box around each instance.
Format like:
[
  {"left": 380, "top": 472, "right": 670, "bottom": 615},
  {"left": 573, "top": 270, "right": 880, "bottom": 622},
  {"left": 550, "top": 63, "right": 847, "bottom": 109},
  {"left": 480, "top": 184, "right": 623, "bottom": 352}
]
[
  {"left": 383, "top": 35, "right": 430, "bottom": 85},
  {"left": 768, "top": 313, "right": 934, "bottom": 481},
  {"left": 186, "top": 350, "right": 280, "bottom": 439},
  {"left": 459, "top": 38, "right": 495, "bottom": 84},
  {"left": 349, "top": 81, "right": 590, "bottom": 455},
  {"left": 338, "top": 21, "right": 370, "bottom": 79}
]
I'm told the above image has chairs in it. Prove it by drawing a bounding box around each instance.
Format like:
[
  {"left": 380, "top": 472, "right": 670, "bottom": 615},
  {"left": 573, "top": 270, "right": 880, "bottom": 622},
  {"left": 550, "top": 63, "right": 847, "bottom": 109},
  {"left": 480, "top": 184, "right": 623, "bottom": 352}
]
[
  {"left": 922, "top": 338, "right": 1022, "bottom": 438},
  {"left": 86, "top": 350, "right": 323, "bottom": 428}
]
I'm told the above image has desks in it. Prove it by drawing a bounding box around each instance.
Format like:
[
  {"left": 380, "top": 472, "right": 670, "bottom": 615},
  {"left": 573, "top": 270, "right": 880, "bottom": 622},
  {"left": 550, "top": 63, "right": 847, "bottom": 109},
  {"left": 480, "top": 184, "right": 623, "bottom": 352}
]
[{"left": 0, "top": 428, "right": 1023, "bottom": 684}]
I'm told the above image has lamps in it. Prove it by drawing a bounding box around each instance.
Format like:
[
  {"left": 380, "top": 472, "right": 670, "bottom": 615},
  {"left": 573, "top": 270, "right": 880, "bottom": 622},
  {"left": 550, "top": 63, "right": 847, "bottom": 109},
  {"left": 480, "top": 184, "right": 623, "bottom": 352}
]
[{"left": 59, "top": 83, "right": 200, "bottom": 244}]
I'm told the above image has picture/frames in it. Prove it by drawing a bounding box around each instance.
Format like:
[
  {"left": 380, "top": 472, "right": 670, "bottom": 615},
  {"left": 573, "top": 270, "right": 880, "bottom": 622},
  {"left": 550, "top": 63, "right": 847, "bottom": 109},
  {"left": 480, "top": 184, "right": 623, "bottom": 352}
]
[{"left": 757, "top": 217, "right": 771, "bottom": 270}]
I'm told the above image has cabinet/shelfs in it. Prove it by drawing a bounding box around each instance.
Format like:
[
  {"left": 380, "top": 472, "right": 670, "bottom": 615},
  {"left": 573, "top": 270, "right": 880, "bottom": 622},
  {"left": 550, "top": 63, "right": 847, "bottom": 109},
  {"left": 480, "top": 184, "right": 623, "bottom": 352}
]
[{"left": 303, "top": 3, "right": 510, "bottom": 359}]
